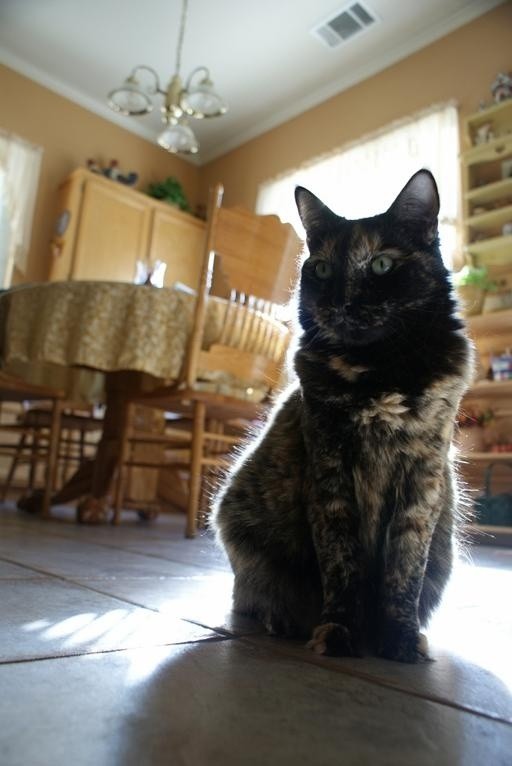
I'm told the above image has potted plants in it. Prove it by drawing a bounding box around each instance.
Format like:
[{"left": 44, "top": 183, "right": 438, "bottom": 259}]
[{"left": 145, "top": 173, "right": 189, "bottom": 211}]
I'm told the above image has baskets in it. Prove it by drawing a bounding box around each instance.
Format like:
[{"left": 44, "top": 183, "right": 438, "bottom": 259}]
[{"left": 474, "top": 462, "right": 512, "bottom": 526}]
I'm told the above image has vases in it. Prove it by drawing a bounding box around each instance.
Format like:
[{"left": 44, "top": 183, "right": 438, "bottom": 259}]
[{"left": 454, "top": 425, "right": 486, "bottom": 452}]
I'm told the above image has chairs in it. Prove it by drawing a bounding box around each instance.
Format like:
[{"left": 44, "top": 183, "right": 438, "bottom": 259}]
[
  {"left": 66, "top": 178, "right": 305, "bottom": 539},
  {"left": 0, "top": 406, "right": 104, "bottom": 520}
]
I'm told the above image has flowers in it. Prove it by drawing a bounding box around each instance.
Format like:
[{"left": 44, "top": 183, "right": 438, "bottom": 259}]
[{"left": 455, "top": 406, "right": 496, "bottom": 429}]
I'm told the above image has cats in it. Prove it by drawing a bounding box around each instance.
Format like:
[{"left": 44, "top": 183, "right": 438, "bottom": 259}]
[{"left": 205, "top": 167, "right": 476, "bottom": 665}]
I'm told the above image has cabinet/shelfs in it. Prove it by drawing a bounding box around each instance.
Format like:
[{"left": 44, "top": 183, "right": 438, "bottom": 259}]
[
  {"left": 39, "top": 161, "right": 215, "bottom": 291},
  {"left": 452, "top": 97, "right": 512, "bottom": 547}
]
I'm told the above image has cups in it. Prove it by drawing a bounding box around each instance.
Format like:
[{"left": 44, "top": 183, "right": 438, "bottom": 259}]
[{"left": 489, "top": 357, "right": 512, "bottom": 381}]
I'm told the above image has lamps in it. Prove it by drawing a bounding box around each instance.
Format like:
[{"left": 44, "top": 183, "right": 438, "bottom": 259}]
[{"left": 102, "top": 0, "right": 231, "bottom": 155}]
